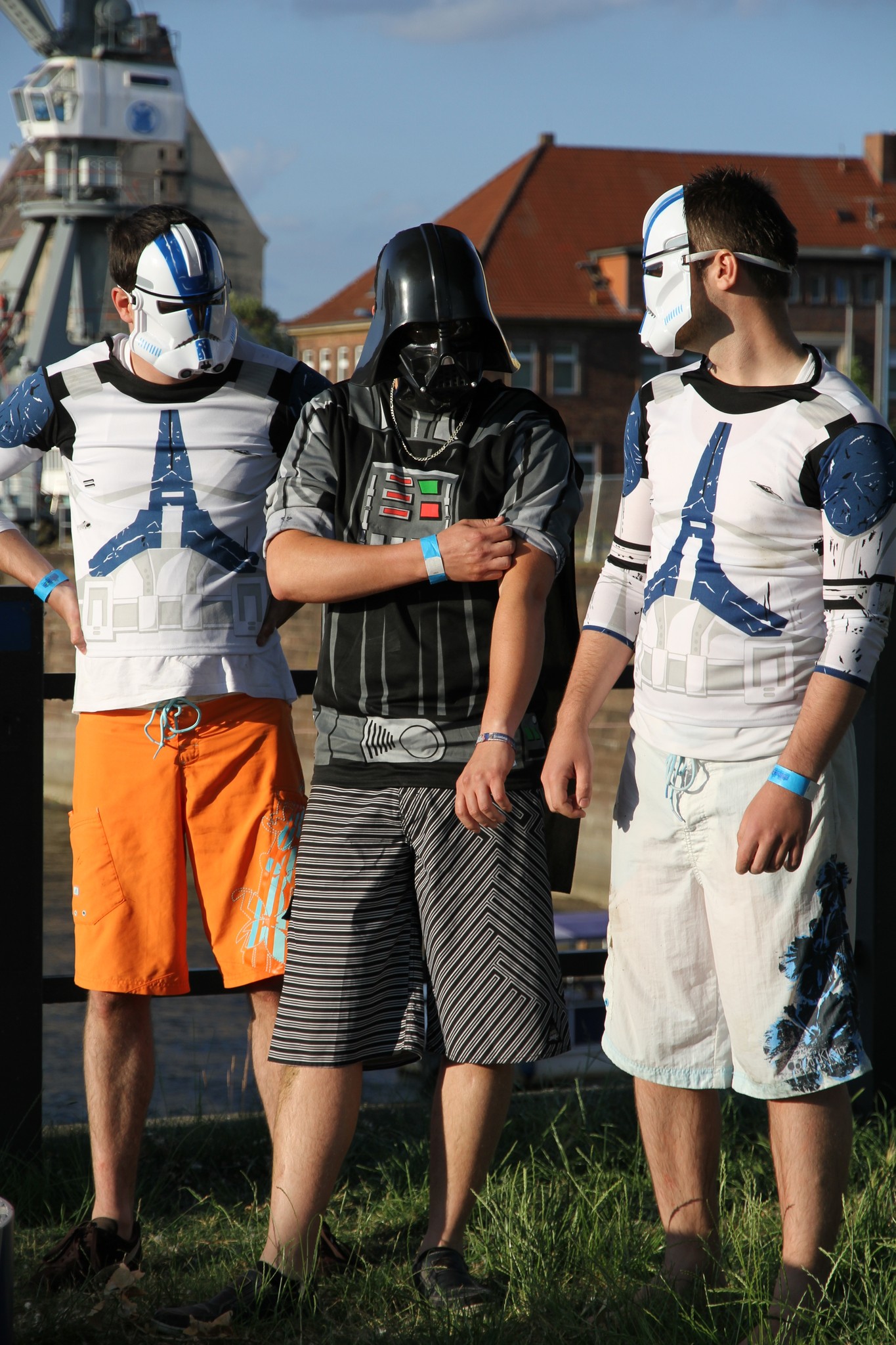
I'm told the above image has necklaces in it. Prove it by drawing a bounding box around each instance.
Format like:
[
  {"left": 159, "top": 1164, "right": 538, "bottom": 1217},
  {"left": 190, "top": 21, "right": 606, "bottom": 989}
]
[{"left": 390, "top": 378, "right": 475, "bottom": 461}]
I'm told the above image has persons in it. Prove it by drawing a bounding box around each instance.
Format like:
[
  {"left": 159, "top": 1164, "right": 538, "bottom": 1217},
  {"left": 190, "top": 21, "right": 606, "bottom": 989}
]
[
  {"left": 0, "top": 203, "right": 360, "bottom": 1292},
  {"left": 149, "top": 224, "right": 586, "bottom": 1336},
  {"left": 541, "top": 165, "right": 896, "bottom": 1344}
]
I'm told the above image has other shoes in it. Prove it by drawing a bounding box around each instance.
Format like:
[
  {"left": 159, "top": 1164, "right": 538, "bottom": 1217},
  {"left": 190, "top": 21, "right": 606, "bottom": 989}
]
[
  {"left": 35, "top": 1216, "right": 142, "bottom": 1295},
  {"left": 411, "top": 1248, "right": 494, "bottom": 1317},
  {"left": 150, "top": 1269, "right": 321, "bottom": 1334},
  {"left": 314, "top": 1220, "right": 363, "bottom": 1271}
]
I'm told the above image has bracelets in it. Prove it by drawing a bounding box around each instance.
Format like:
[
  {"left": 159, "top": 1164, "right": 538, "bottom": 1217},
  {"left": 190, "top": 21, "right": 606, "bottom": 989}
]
[
  {"left": 419, "top": 533, "right": 449, "bottom": 585},
  {"left": 476, "top": 733, "right": 516, "bottom": 748},
  {"left": 766, "top": 764, "right": 822, "bottom": 802},
  {"left": 34, "top": 570, "right": 70, "bottom": 602}
]
